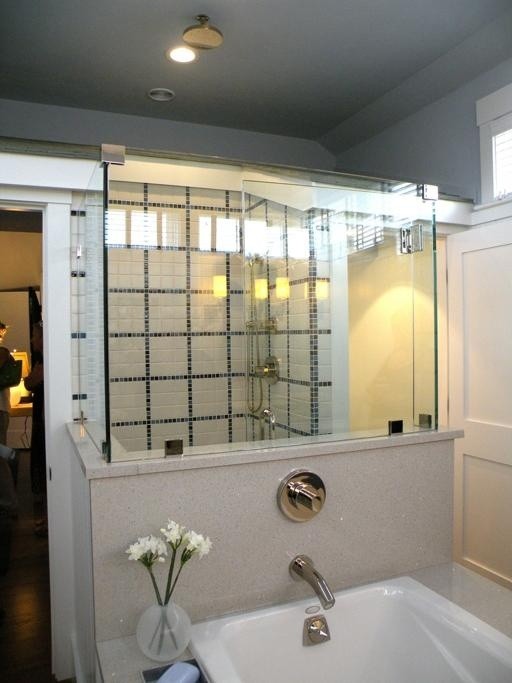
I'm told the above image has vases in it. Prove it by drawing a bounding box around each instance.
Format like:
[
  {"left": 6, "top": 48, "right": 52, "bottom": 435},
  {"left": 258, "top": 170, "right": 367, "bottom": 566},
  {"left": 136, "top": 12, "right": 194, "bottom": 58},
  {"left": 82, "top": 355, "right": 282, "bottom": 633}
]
[{"left": 136, "top": 603, "right": 192, "bottom": 661}]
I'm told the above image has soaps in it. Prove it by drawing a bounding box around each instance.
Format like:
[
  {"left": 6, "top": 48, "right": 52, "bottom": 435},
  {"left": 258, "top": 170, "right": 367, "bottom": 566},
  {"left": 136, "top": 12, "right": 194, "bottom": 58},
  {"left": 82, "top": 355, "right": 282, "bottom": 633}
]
[{"left": 155, "top": 661, "right": 199, "bottom": 683}]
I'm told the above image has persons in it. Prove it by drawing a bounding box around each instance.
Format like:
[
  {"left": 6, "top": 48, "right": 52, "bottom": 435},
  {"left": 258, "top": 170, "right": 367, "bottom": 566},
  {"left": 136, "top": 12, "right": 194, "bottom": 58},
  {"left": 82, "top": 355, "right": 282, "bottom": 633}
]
[
  {"left": 0, "top": 320, "right": 22, "bottom": 510},
  {"left": 20, "top": 320, "right": 47, "bottom": 537}
]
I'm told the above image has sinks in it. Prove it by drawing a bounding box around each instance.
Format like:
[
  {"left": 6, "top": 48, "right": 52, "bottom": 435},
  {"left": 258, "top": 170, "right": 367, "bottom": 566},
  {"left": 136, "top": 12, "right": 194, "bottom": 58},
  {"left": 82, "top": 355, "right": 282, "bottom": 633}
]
[{"left": 190, "top": 574, "right": 512, "bottom": 683}]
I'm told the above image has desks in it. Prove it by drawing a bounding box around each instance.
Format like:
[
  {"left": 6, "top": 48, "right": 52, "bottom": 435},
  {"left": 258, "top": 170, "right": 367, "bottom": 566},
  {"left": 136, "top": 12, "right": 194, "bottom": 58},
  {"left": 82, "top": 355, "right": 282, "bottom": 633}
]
[{"left": 0, "top": 383, "right": 34, "bottom": 449}]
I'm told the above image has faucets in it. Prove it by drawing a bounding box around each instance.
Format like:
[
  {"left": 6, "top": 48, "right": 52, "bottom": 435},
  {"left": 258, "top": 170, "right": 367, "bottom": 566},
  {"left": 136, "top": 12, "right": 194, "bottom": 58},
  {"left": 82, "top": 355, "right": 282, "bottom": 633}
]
[{"left": 288, "top": 554, "right": 336, "bottom": 610}]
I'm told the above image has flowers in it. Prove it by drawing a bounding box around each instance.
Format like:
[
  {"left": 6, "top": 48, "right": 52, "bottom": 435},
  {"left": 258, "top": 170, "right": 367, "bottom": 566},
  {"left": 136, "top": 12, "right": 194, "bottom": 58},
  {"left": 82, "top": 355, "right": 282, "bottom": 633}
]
[{"left": 126, "top": 518, "right": 212, "bottom": 657}]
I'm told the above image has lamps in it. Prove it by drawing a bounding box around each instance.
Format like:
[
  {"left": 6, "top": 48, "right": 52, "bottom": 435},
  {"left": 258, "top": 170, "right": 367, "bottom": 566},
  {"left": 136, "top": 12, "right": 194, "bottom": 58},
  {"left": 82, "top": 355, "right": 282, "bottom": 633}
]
[
  {"left": 212, "top": 275, "right": 330, "bottom": 302},
  {"left": 183, "top": 15, "right": 223, "bottom": 50}
]
